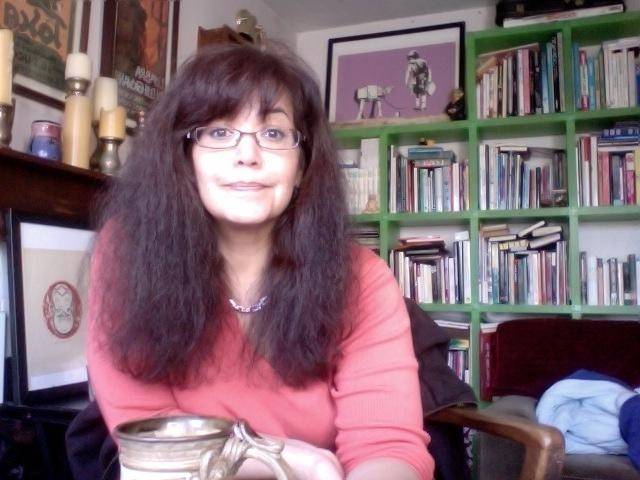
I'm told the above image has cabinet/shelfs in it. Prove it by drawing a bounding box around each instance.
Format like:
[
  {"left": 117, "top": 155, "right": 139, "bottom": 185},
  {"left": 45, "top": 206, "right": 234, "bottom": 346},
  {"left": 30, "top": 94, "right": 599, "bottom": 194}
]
[{"left": 328, "top": 9, "right": 639, "bottom": 480}]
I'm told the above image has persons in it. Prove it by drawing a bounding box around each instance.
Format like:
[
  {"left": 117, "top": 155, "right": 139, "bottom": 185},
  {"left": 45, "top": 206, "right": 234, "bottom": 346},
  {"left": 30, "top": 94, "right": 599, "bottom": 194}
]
[{"left": 71, "top": 40, "right": 435, "bottom": 480}]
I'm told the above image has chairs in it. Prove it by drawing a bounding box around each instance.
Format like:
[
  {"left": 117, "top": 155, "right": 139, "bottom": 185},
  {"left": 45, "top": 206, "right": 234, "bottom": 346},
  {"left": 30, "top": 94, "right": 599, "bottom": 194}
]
[{"left": 425, "top": 404, "right": 566, "bottom": 479}]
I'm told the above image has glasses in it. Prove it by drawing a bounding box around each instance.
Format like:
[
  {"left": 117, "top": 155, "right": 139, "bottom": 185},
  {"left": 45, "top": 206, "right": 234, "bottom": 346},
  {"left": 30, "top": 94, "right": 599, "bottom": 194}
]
[{"left": 186, "top": 124, "right": 305, "bottom": 149}]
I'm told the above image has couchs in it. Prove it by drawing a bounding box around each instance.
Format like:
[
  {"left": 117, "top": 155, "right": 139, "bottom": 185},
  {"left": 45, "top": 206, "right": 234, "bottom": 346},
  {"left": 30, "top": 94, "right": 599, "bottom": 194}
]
[{"left": 479, "top": 316, "right": 640, "bottom": 480}]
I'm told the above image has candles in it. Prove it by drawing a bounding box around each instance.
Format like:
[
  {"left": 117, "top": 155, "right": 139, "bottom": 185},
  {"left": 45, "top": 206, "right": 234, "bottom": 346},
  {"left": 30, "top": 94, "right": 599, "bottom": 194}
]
[
  {"left": 0, "top": 29, "right": 15, "bottom": 106},
  {"left": 92, "top": 76, "right": 118, "bottom": 121},
  {"left": 98, "top": 106, "right": 126, "bottom": 139},
  {"left": 62, "top": 95, "right": 90, "bottom": 170},
  {"left": 65, "top": 54, "right": 91, "bottom": 81}
]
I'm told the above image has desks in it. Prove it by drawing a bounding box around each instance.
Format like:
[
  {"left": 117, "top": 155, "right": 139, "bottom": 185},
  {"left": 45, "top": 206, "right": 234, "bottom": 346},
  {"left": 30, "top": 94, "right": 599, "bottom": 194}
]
[{"left": 0, "top": 396, "right": 92, "bottom": 480}]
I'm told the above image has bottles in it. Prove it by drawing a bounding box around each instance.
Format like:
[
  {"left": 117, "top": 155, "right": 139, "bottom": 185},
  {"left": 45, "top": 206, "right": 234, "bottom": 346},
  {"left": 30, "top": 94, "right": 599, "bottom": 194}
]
[{"left": 27, "top": 120, "right": 60, "bottom": 160}]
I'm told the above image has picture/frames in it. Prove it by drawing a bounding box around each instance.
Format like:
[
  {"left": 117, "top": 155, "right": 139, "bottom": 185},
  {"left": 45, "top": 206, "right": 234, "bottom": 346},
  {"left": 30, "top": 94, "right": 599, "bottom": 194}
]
[
  {"left": 324, "top": 20, "right": 465, "bottom": 122},
  {"left": 100, "top": 0, "right": 179, "bottom": 121},
  {"left": 0, "top": 0, "right": 91, "bottom": 112}
]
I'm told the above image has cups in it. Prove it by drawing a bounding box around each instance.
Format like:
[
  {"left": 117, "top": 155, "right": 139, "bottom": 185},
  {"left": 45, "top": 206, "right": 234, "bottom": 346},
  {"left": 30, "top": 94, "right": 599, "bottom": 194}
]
[{"left": 112, "top": 413, "right": 290, "bottom": 480}]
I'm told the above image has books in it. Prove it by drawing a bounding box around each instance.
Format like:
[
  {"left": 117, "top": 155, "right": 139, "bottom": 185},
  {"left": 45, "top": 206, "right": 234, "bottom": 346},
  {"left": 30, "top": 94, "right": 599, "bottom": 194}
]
[
  {"left": 335, "top": 137, "right": 639, "bottom": 215},
  {"left": 342, "top": 221, "right": 639, "bottom": 306},
  {"left": 434, "top": 320, "right": 497, "bottom": 402},
  {"left": 477, "top": 33, "right": 639, "bottom": 118}
]
